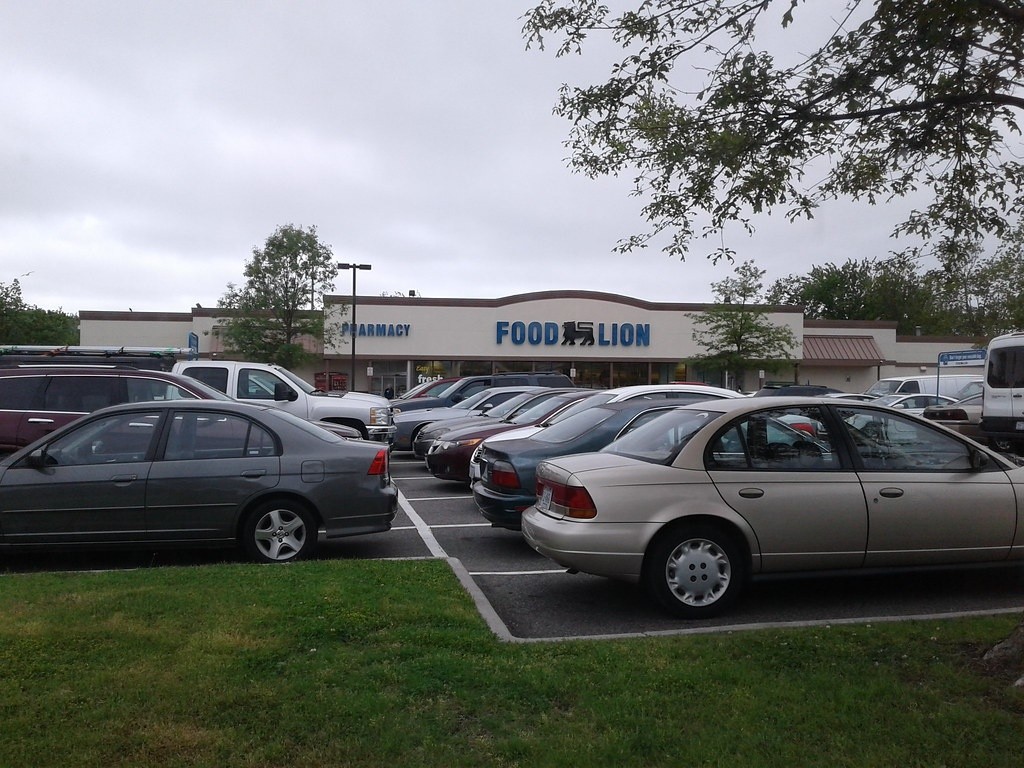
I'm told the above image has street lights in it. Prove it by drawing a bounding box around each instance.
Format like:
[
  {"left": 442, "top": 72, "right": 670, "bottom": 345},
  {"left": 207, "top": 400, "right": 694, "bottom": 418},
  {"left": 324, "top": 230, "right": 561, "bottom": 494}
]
[{"left": 336, "top": 262, "right": 372, "bottom": 392}]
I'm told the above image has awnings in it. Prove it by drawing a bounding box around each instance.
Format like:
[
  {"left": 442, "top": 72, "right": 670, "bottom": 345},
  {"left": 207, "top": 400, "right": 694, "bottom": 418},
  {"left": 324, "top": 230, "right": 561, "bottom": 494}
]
[{"left": 803, "top": 335, "right": 886, "bottom": 364}]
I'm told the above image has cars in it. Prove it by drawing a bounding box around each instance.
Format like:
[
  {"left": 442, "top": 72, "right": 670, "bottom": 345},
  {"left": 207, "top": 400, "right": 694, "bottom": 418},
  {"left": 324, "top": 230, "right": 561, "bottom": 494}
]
[
  {"left": 0, "top": 398, "right": 400, "bottom": 567},
  {"left": 389, "top": 369, "right": 990, "bottom": 490},
  {"left": 519, "top": 394, "right": 1024, "bottom": 620},
  {"left": 469, "top": 398, "right": 835, "bottom": 534}
]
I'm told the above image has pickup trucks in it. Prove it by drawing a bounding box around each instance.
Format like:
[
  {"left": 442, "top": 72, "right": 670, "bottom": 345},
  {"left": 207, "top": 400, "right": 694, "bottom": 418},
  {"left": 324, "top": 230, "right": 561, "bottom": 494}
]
[{"left": 155, "top": 359, "right": 399, "bottom": 453}]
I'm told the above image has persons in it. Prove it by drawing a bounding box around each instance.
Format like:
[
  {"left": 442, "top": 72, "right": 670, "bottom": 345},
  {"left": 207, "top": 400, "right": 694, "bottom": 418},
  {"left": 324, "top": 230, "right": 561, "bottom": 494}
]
[{"left": 384, "top": 384, "right": 394, "bottom": 400}]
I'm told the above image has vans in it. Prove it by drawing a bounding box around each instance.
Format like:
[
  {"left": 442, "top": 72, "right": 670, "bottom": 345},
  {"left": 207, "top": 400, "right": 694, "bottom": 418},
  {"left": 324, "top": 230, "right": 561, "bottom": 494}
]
[{"left": 977, "top": 330, "right": 1024, "bottom": 454}]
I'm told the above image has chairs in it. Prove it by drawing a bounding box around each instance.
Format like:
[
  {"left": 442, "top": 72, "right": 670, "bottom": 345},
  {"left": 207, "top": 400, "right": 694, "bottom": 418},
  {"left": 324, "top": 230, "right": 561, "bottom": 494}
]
[{"left": 764, "top": 441, "right": 828, "bottom": 471}]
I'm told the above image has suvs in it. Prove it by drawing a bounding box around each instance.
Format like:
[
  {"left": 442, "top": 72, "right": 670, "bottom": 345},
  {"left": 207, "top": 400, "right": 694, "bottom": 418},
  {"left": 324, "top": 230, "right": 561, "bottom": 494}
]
[{"left": 0, "top": 344, "right": 364, "bottom": 461}]
